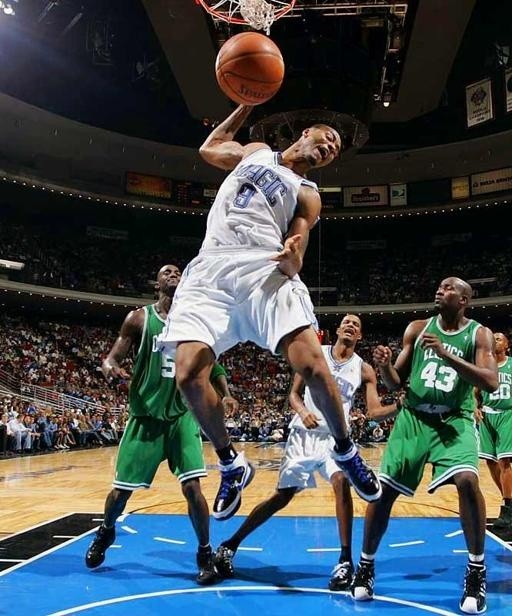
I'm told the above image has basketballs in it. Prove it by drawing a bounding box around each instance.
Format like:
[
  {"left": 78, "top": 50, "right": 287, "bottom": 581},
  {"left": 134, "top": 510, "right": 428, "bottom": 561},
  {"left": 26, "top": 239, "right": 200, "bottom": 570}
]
[{"left": 214, "top": 31, "right": 284, "bottom": 106}]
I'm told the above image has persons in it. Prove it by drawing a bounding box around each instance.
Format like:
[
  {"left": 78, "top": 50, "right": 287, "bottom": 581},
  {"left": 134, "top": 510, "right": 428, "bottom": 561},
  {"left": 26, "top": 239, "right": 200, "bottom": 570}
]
[
  {"left": 83, "top": 265, "right": 220, "bottom": 585},
  {"left": 155, "top": 101, "right": 391, "bottom": 521},
  {"left": 211, "top": 307, "right": 405, "bottom": 591},
  {"left": 348, "top": 271, "right": 498, "bottom": 613},
  {"left": 0, "top": 181, "right": 512, "bottom": 455},
  {"left": 473, "top": 328, "right": 512, "bottom": 535}
]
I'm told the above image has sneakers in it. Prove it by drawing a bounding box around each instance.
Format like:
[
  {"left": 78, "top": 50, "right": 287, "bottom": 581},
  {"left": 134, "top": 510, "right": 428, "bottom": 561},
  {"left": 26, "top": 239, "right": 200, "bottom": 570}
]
[
  {"left": 493, "top": 506, "right": 510, "bottom": 526},
  {"left": 212, "top": 451, "right": 254, "bottom": 519},
  {"left": 460, "top": 564, "right": 487, "bottom": 614},
  {"left": 330, "top": 560, "right": 374, "bottom": 601},
  {"left": 86, "top": 525, "right": 115, "bottom": 568},
  {"left": 197, "top": 543, "right": 233, "bottom": 584},
  {"left": 332, "top": 441, "right": 383, "bottom": 503}
]
